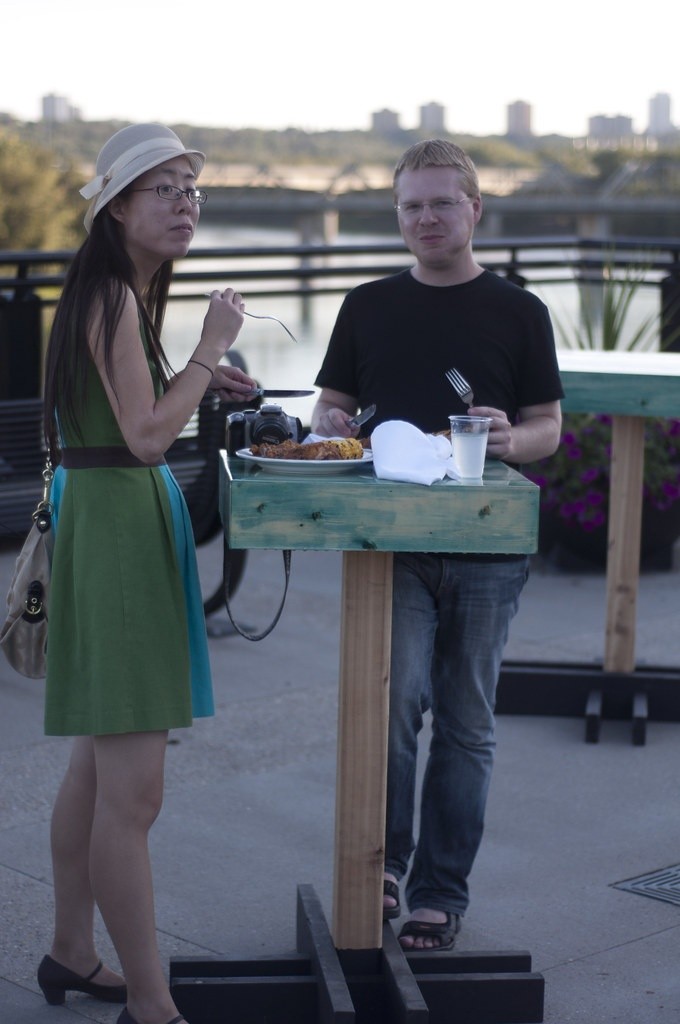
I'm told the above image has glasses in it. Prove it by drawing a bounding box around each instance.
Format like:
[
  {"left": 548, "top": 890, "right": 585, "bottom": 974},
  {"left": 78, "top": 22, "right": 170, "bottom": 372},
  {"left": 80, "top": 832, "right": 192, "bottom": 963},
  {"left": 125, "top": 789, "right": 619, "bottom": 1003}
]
[
  {"left": 393, "top": 196, "right": 476, "bottom": 216},
  {"left": 127, "top": 185, "right": 208, "bottom": 204}
]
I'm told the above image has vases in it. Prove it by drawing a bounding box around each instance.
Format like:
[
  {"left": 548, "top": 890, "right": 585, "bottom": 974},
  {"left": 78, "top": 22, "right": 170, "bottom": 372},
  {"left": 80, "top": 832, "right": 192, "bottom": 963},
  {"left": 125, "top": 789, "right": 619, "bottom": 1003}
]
[{"left": 539, "top": 510, "right": 675, "bottom": 572}]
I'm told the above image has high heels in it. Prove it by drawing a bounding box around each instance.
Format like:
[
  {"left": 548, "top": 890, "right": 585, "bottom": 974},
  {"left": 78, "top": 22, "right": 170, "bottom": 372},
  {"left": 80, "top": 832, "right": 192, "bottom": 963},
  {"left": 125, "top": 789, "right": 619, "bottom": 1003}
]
[
  {"left": 37, "top": 954, "right": 129, "bottom": 1006},
  {"left": 117, "top": 1007, "right": 183, "bottom": 1024}
]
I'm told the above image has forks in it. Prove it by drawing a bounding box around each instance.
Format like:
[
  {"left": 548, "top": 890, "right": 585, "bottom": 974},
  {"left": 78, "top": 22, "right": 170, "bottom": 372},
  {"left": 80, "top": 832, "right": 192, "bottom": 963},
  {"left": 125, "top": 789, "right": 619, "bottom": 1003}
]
[
  {"left": 244, "top": 312, "right": 297, "bottom": 343},
  {"left": 444, "top": 367, "right": 473, "bottom": 408}
]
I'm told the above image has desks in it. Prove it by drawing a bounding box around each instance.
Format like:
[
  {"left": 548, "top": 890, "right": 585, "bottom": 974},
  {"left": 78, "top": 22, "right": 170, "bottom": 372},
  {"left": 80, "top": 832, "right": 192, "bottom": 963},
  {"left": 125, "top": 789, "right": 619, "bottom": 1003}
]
[
  {"left": 148, "top": 447, "right": 546, "bottom": 1024},
  {"left": 484, "top": 353, "right": 680, "bottom": 741}
]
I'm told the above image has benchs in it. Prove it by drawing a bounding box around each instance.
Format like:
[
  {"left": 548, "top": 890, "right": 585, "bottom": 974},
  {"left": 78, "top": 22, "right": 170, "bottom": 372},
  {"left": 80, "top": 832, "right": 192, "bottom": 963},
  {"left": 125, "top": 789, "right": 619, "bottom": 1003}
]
[{"left": 0, "top": 350, "right": 255, "bottom": 616}]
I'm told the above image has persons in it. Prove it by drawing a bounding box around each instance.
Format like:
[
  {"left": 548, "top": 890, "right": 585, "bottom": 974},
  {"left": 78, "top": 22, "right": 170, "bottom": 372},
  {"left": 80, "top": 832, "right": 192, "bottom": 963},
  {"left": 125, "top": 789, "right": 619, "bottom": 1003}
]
[
  {"left": 39, "top": 124, "right": 260, "bottom": 1024},
  {"left": 311, "top": 140, "right": 565, "bottom": 950}
]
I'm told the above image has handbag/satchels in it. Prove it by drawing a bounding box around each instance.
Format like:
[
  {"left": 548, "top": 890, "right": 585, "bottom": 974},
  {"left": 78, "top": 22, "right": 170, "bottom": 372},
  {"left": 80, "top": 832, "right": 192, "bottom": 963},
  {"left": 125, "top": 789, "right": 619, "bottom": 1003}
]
[{"left": 0, "top": 501, "right": 55, "bottom": 678}]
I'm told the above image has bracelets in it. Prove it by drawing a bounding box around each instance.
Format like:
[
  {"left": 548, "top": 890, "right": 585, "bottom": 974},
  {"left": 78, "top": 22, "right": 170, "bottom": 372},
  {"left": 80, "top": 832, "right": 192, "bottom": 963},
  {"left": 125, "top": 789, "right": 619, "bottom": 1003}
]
[{"left": 185, "top": 360, "right": 217, "bottom": 377}]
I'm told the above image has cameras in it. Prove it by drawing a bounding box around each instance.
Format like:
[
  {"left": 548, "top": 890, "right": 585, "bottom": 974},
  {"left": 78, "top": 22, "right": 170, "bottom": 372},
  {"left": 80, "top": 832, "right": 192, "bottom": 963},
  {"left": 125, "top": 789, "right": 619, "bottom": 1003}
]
[{"left": 225, "top": 403, "right": 303, "bottom": 458}]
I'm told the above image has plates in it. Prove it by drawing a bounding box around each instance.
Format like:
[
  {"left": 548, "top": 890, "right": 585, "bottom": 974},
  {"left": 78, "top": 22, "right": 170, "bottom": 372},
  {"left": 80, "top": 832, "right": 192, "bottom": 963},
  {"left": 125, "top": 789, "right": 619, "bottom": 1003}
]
[{"left": 235, "top": 448, "right": 375, "bottom": 474}]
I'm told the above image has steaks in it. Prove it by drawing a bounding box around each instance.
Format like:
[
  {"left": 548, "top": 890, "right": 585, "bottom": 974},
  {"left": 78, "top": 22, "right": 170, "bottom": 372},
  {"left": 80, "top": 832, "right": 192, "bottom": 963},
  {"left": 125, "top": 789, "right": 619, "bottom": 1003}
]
[{"left": 251, "top": 439, "right": 343, "bottom": 460}]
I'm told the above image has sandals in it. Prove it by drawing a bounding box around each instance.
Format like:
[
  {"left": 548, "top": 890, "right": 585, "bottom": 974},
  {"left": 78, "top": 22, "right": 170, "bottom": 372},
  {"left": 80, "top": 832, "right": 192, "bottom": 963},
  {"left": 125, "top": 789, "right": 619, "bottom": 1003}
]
[
  {"left": 396, "top": 907, "right": 461, "bottom": 951},
  {"left": 382, "top": 874, "right": 401, "bottom": 918}
]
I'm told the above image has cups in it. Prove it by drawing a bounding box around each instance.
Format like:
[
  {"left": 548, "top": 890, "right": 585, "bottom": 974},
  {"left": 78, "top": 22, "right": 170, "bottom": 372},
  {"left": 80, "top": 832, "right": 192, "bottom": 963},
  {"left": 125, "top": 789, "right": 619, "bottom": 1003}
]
[{"left": 448, "top": 415, "right": 494, "bottom": 479}]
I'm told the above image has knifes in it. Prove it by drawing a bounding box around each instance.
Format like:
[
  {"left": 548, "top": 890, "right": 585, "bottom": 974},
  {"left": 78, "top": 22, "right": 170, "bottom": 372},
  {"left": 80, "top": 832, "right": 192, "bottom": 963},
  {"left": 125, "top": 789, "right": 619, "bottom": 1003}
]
[
  {"left": 244, "top": 390, "right": 316, "bottom": 398},
  {"left": 348, "top": 404, "right": 376, "bottom": 430}
]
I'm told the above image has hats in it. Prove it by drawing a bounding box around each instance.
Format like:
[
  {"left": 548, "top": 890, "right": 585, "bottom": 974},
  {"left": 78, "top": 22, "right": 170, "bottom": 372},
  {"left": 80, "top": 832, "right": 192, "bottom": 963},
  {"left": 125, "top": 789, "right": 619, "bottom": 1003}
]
[{"left": 79, "top": 123, "right": 207, "bottom": 234}]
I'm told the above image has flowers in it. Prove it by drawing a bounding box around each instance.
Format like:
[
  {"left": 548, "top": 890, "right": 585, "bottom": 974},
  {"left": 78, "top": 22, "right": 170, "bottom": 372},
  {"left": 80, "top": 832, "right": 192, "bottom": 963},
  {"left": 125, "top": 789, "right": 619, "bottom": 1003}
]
[{"left": 521, "top": 231, "right": 680, "bottom": 536}]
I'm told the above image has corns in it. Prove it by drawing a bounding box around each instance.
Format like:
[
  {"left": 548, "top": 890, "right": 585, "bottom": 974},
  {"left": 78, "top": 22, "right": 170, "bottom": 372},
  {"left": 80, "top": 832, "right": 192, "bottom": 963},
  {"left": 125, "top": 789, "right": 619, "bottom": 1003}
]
[{"left": 324, "top": 438, "right": 364, "bottom": 460}]
